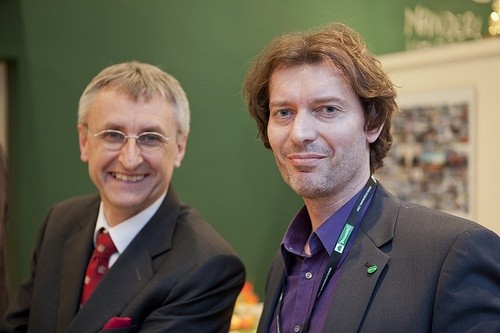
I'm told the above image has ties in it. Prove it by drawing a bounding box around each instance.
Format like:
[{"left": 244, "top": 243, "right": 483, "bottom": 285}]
[{"left": 79, "top": 226, "right": 118, "bottom": 311}]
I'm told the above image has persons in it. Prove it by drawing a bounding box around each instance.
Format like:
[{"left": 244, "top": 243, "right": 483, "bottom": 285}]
[
  {"left": 242, "top": 23, "right": 500, "bottom": 333},
  {"left": 0, "top": 60, "right": 247, "bottom": 333}
]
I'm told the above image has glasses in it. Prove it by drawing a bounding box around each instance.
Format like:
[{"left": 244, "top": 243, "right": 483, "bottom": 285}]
[{"left": 87, "top": 129, "right": 180, "bottom": 151}]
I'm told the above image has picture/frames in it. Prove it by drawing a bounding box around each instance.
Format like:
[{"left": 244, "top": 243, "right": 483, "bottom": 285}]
[{"left": 375, "top": 87, "right": 475, "bottom": 221}]
